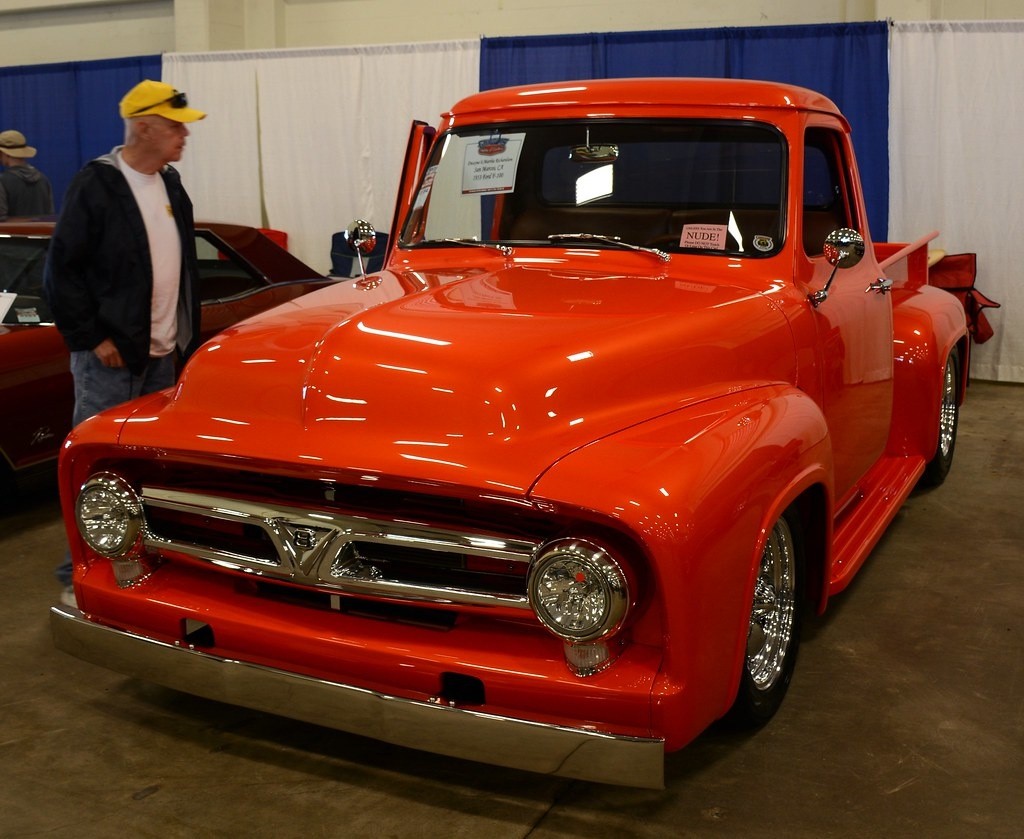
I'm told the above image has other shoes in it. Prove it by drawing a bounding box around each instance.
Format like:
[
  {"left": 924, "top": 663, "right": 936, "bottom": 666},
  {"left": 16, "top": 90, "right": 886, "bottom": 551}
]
[{"left": 53, "top": 560, "right": 73, "bottom": 583}]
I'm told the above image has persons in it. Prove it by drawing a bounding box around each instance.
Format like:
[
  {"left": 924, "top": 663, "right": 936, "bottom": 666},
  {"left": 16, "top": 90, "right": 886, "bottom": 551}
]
[
  {"left": 0, "top": 130, "right": 55, "bottom": 290},
  {"left": 40, "top": 78, "right": 207, "bottom": 611}
]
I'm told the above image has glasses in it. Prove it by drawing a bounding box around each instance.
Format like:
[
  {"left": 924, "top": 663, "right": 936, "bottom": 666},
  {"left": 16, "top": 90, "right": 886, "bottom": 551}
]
[{"left": 130, "top": 92, "right": 187, "bottom": 114}]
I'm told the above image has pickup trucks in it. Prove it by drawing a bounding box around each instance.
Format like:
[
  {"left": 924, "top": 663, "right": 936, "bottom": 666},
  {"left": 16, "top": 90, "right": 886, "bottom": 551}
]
[{"left": 49, "top": 76, "right": 1002, "bottom": 791}]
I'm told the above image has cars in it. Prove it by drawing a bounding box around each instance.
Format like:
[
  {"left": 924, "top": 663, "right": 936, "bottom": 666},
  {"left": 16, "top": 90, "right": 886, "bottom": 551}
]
[{"left": 1, "top": 220, "right": 336, "bottom": 514}]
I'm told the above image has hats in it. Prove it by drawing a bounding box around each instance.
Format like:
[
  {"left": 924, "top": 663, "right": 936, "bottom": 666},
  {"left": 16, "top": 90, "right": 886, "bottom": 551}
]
[
  {"left": 0, "top": 130, "right": 36, "bottom": 158},
  {"left": 118, "top": 78, "right": 208, "bottom": 124}
]
